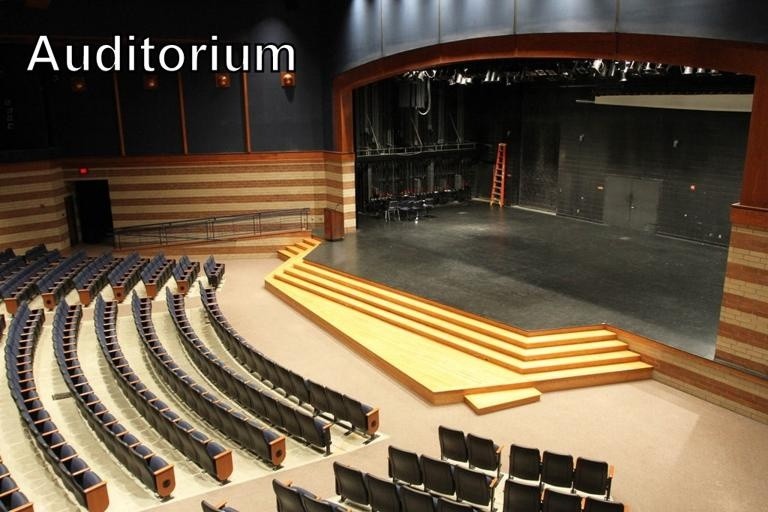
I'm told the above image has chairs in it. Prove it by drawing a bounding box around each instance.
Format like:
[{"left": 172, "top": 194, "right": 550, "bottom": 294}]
[
  {"left": 383, "top": 197, "right": 434, "bottom": 224},
  {"left": 0, "top": 241, "right": 625, "bottom": 512}
]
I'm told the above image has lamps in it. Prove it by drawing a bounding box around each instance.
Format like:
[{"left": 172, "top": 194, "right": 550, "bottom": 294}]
[{"left": 402, "top": 58, "right": 721, "bottom": 86}]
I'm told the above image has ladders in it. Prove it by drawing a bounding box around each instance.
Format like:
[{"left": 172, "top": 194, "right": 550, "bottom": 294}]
[{"left": 489, "top": 143, "right": 506, "bottom": 208}]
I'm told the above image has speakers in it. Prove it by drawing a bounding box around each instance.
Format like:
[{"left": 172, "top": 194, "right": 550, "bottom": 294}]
[{"left": 64, "top": 180, "right": 116, "bottom": 250}]
